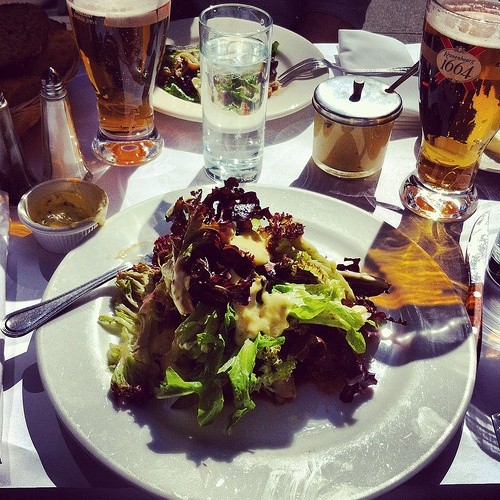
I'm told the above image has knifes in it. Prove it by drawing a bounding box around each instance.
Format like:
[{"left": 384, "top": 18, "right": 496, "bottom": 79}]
[{"left": 466, "top": 209, "right": 492, "bottom": 336}]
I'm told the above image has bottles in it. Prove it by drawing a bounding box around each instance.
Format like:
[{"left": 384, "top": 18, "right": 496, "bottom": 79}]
[
  {"left": 38, "top": 66, "right": 93, "bottom": 180},
  {"left": 0, "top": 91, "right": 39, "bottom": 204}
]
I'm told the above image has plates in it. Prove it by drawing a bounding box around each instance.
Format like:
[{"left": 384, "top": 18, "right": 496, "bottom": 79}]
[
  {"left": 35, "top": 183, "right": 478, "bottom": 499},
  {"left": 152, "top": 12, "right": 330, "bottom": 124}
]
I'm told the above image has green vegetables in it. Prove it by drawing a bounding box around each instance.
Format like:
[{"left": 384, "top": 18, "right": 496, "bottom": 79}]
[
  {"left": 97, "top": 176, "right": 408, "bottom": 441},
  {"left": 160, "top": 41, "right": 281, "bottom": 113}
]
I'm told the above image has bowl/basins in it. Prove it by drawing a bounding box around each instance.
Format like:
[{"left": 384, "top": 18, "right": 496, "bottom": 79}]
[{"left": 17, "top": 177, "right": 109, "bottom": 253}]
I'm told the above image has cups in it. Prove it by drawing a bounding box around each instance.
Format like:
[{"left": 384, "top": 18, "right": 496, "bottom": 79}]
[
  {"left": 399, "top": 0, "right": 500, "bottom": 224},
  {"left": 198, "top": 3, "right": 274, "bottom": 186},
  {"left": 311, "top": 73, "right": 404, "bottom": 185},
  {"left": 63, "top": 1, "right": 174, "bottom": 170},
  {"left": 486, "top": 228, "right": 500, "bottom": 289}
]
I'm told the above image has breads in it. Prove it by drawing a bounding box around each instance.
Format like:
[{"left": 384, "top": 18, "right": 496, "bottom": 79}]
[{"left": 0, "top": 1, "right": 79, "bottom": 110}]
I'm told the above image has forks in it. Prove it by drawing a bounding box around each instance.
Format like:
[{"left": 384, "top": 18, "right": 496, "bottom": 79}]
[{"left": 278, "top": 57, "right": 418, "bottom": 87}]
